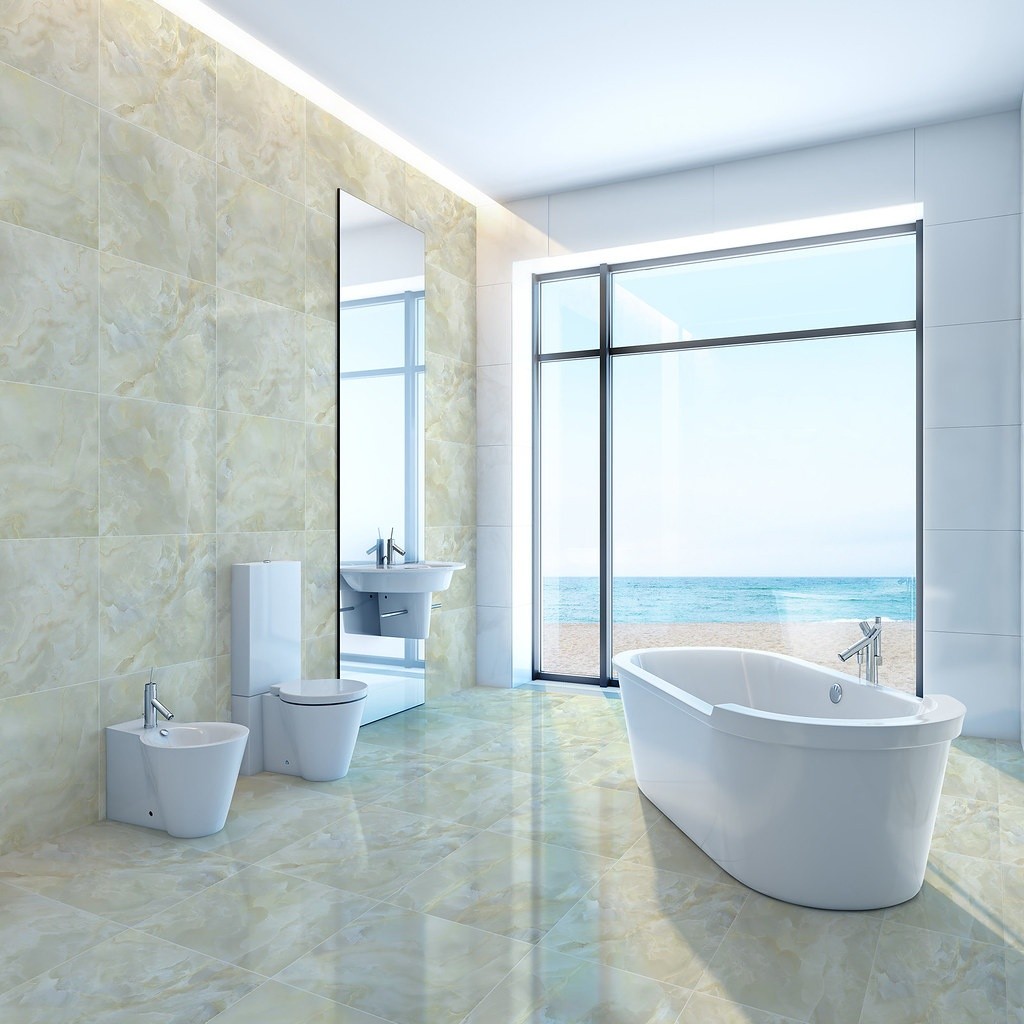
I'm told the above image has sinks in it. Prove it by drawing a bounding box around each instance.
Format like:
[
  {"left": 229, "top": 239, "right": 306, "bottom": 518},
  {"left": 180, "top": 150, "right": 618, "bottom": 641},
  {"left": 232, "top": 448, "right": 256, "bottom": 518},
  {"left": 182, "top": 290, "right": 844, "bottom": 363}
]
[
  {"left": 340, "top": 559, "right": 469, "bottom": 596},
  {"left": 143, "top": 719, "right": 254, "bottom": 838}
]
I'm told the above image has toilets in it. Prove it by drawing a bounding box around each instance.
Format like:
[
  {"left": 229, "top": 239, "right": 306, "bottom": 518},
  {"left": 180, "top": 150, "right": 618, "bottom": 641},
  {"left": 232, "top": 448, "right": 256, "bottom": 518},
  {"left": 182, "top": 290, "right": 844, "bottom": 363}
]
[{"left": 259, "top": 673, "right": 370, "bottom": 785}]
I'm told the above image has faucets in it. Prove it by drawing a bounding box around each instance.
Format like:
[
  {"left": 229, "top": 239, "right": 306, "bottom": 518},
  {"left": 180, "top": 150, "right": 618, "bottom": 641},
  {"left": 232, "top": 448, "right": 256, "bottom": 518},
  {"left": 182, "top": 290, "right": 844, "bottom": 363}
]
[
  {"left": 144, "top": 681, "right": 176, "bottom": 727},
  {"left": 387, "top": 538, "right": 405, "bottom": 564},
  {"left": 837, "top": 621, "right": 880, "bottom": 663},
  {"left": 366, "top": 537, "right": 386, "bottom": 566}
]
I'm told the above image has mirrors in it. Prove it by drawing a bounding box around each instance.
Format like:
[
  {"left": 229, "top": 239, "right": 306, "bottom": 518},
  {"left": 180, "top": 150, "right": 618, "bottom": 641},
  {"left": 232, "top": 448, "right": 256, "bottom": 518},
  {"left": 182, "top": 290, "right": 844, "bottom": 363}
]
[{"left": 336, "top": 188, "right": 427, "bottom": 730}]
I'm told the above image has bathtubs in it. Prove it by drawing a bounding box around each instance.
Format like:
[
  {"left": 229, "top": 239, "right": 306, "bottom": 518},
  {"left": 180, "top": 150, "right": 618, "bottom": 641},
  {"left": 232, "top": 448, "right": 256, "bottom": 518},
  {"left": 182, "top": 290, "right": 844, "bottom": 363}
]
[{"left": 608, "top": 644, "right": 968, "bottom": 911}]
